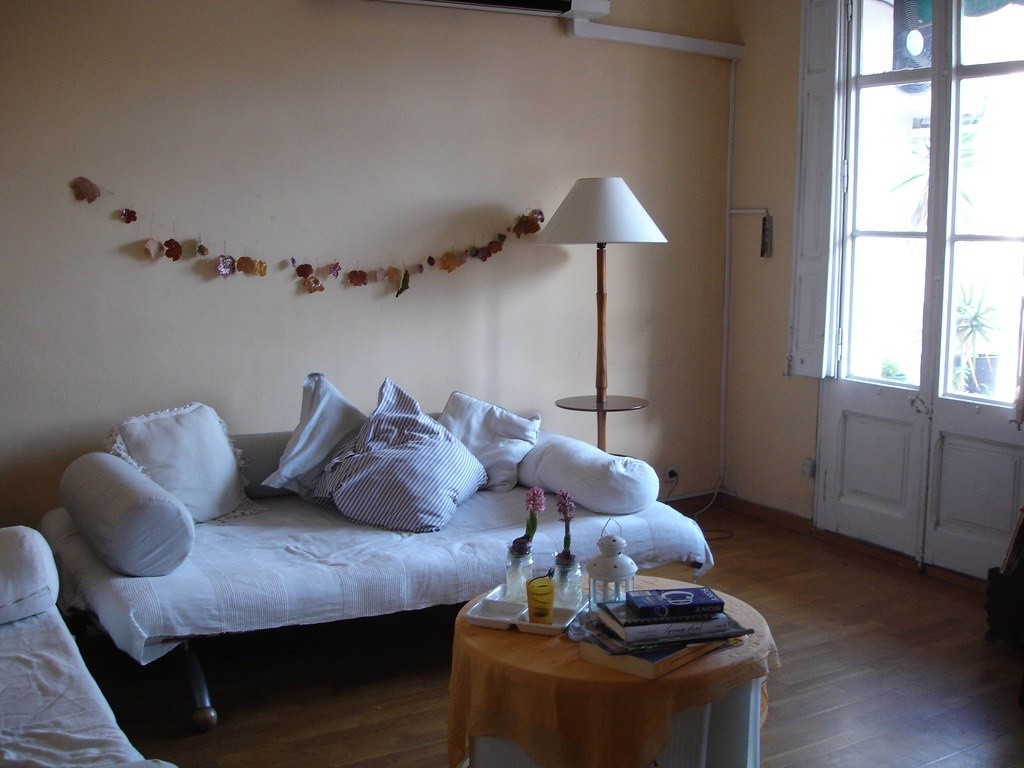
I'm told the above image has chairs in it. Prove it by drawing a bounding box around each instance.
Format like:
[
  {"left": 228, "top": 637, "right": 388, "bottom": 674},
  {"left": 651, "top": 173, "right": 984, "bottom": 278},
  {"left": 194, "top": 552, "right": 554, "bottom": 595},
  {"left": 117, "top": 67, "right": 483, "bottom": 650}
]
[{"left": 0, "top": 524, "right": 181, "bottom": 768}]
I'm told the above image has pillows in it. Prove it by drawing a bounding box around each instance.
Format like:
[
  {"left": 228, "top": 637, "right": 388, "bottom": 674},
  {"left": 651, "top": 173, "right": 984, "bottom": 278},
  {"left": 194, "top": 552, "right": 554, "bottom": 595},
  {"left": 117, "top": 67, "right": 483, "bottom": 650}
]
[
  {"left": 436, "top": 391, "right": 544, "bottom": 494},
  {"left": 312, "top": 375, "right": 488, "bottom": 534},
  {"left": 102, "top": 402, "right": 269, "bottom": 525},
  {"left": 259, "top": 369, "right": 368, "bottom": 500}
]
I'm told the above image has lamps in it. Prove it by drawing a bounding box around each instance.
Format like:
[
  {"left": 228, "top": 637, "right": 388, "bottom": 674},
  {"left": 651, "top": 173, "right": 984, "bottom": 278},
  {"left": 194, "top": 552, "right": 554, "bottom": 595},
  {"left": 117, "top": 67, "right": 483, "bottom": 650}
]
[{"left": 530, "top": 177, "right": 669, "bottom": 403}]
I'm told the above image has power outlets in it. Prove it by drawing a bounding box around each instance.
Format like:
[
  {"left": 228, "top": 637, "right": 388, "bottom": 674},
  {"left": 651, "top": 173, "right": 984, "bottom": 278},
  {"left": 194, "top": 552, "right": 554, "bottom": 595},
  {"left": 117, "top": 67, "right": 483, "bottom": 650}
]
[{"left": 664, "top": 463, "right": 680, "bottom": 482}]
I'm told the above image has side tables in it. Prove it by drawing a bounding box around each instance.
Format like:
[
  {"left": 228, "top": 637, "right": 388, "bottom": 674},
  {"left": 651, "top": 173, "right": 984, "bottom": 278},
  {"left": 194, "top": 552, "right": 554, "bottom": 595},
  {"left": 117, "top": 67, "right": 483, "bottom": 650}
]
[{"left": 555, "top": 395, "right": 650, "bottom": 452}]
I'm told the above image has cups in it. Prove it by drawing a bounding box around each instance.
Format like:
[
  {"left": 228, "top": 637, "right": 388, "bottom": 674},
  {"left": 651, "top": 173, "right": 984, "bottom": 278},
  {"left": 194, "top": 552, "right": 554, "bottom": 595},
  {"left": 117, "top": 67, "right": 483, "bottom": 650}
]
[{"left": 526, "top": 578, "right": 555, "bottom": 625}]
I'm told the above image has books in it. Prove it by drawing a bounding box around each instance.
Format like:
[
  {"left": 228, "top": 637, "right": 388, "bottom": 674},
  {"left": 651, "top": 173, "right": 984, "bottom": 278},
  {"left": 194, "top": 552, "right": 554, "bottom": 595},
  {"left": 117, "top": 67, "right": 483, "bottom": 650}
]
[{"left": 576, "top": 586, "right": 755, "bottom": 681}]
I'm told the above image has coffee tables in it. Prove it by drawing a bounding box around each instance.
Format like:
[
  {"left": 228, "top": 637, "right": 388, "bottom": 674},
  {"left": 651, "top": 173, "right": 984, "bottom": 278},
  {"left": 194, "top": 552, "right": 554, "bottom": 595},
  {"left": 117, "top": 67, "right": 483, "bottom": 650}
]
[{"left": 446, "top": 570, "right": 782, "bottom": 768}]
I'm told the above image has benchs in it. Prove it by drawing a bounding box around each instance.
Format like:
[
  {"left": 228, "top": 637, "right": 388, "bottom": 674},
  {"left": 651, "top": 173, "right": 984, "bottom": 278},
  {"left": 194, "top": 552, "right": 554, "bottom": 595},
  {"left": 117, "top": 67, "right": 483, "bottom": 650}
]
[{"left": 40, "top": 412, "right": 716, "bottom": 734}]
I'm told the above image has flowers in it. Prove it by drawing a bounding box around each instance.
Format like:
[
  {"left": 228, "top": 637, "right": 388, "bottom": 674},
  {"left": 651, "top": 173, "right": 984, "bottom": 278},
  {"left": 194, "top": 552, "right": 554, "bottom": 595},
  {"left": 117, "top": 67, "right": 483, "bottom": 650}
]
[
  {"left": 553, "top": 486, "right": 576, "bottom": 591},
  {"left": 508, "top": 485, "right": 546, "bottom": 568}
]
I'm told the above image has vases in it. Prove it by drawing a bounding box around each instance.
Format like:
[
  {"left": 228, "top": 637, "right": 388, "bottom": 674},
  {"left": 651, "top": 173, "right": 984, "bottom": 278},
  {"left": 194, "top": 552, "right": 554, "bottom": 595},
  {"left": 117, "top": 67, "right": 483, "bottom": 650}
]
[
  {"left": 504, "top": 554, "right": 535, "bottom": 604},
  {"left": 549, "top": 564, "right": 585, "bottom": 611}
]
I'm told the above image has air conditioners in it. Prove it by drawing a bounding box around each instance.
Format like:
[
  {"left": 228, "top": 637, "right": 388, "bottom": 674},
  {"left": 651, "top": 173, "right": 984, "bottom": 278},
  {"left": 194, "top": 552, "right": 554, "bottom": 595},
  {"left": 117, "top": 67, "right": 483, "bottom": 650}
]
[{"left": 371, "top": 0, "right": 611, "bottom": 20}]
